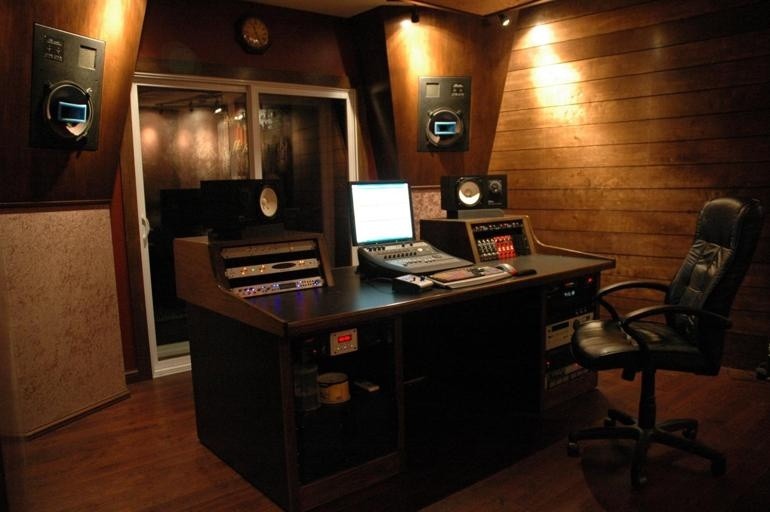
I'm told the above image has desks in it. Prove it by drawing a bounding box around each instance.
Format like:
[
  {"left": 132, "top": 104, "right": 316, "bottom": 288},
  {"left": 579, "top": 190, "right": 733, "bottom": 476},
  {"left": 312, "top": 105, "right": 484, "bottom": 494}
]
[{"left": 173, "top": 213, "right": 617, "bottom": 512}]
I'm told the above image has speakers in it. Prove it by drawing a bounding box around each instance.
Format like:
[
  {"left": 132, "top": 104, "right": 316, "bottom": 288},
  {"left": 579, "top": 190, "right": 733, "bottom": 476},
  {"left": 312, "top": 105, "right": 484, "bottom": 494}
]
[
  {"left": 440, "top": 174, "right": 508, "bottom": 212},
  {"left": 198, "top": 179, "right": 287, "bottom": 230},
  {"left": 28, "top": 23, "right": 106, "bottom": 152},
  {"left": 417, "top": 76, "right": 472, "bottom": 153}
]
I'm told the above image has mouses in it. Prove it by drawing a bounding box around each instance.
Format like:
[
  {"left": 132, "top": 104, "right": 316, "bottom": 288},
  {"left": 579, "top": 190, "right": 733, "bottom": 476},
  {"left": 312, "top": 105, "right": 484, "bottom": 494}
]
[{"left": 496, "top": 263, "right": 518, "bottom": 275}]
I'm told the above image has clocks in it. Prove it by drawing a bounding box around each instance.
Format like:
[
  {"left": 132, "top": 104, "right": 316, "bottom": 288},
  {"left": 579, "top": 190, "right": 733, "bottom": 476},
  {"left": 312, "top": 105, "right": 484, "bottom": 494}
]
[{"left": 235, "top": 12, "right": 273, "bottom": 55}]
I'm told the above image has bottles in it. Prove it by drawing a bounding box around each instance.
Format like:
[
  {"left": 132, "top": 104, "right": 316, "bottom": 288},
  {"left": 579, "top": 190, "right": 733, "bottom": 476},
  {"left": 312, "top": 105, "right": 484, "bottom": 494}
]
[{"left": 291, "top": 362, "right": 322, "bottom": 414}]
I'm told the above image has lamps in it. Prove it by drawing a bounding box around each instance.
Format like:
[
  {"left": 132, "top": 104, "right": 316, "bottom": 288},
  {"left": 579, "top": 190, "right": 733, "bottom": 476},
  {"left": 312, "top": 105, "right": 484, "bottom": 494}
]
[
  {"left": 410, "top": 6, "right": 421, "bottom": 22},
  {"left": 213, "top": 95, "right": 223, "bottom": 114},
  {"left": 497, "top": 12, "right": 512, "bottom": 28}
]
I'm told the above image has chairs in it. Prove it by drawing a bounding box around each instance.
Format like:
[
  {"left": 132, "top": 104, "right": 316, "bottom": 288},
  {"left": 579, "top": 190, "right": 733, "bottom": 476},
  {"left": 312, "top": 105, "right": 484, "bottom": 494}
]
[{"left": 566, "top": 194, "right": 763, "bottom": 512}]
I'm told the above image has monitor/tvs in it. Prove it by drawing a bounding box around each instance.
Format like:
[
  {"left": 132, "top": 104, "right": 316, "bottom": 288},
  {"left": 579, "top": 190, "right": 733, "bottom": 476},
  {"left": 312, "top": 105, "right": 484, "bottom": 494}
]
[{"left": 348, "top": 179, "right": 415, "bottom": 244}]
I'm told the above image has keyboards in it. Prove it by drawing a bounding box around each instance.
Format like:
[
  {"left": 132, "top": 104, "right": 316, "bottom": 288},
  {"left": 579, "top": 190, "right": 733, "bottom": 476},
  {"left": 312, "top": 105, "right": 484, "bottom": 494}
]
[{"left": 427, "top": 266, "right": 511, "bottom": 289}]
